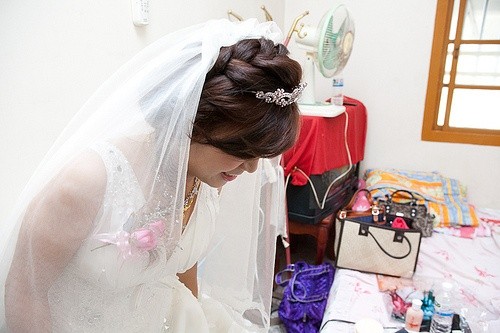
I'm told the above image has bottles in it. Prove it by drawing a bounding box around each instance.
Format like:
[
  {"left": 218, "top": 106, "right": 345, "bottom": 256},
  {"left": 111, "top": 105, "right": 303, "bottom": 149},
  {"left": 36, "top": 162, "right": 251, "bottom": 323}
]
[
  {"left": 331, "top": 76, "right": 344, "bottom": 107},
  {"left": 405, "top": 299, "right": 424, "bottom": 333},
  {"left": 429, "top": 281, "right": 454, "bottom": 333}
]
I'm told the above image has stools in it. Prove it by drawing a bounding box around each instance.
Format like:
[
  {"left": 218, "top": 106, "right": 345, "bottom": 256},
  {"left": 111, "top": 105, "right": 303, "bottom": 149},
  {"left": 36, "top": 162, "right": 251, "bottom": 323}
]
[{"left": 280, "top": 208, "right": 338, "bottom": 264}]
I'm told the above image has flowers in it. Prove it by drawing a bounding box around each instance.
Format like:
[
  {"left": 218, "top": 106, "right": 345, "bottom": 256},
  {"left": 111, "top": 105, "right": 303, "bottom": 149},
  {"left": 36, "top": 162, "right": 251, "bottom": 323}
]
[{"left": 88, "top": 220, "right": 165, "bottom": 275}]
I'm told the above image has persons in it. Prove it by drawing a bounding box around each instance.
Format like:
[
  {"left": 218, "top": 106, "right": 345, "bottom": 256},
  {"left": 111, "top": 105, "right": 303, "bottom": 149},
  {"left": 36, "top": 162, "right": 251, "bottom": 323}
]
[{"left": 0, "top": 18, "right": 308, "bottom": 333}]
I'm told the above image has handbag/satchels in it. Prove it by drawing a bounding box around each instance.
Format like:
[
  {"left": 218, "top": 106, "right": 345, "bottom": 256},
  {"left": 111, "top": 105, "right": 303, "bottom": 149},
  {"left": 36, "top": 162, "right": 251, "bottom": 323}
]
[
  {"left": 333, "top": 212, "right": 423, "bottom": 279},
  {"left": 275, "top": 261, "right": 336, "bottom": 333},
  {"left": 338, "top": 187, "right": 436, "bottom": 238}
]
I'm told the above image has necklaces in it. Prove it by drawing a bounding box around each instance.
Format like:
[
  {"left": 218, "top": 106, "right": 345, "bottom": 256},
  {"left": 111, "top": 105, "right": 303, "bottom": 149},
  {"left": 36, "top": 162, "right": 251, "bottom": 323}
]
[{"left": 136, "top": 134, "right": 200, "bottom": 223}]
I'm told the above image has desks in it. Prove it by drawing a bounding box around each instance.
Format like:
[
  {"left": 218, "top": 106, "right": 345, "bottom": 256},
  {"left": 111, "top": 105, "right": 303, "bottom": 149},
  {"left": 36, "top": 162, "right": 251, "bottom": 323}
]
[{"left": 318, "top": 208, "right": 500, "bottom": 333}]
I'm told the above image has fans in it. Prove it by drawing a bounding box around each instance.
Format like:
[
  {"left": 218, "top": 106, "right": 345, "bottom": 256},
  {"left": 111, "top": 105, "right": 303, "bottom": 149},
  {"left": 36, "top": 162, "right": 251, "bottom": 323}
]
[{"left": 294, "top": 3, "right": 355, "bottom": 119}]
